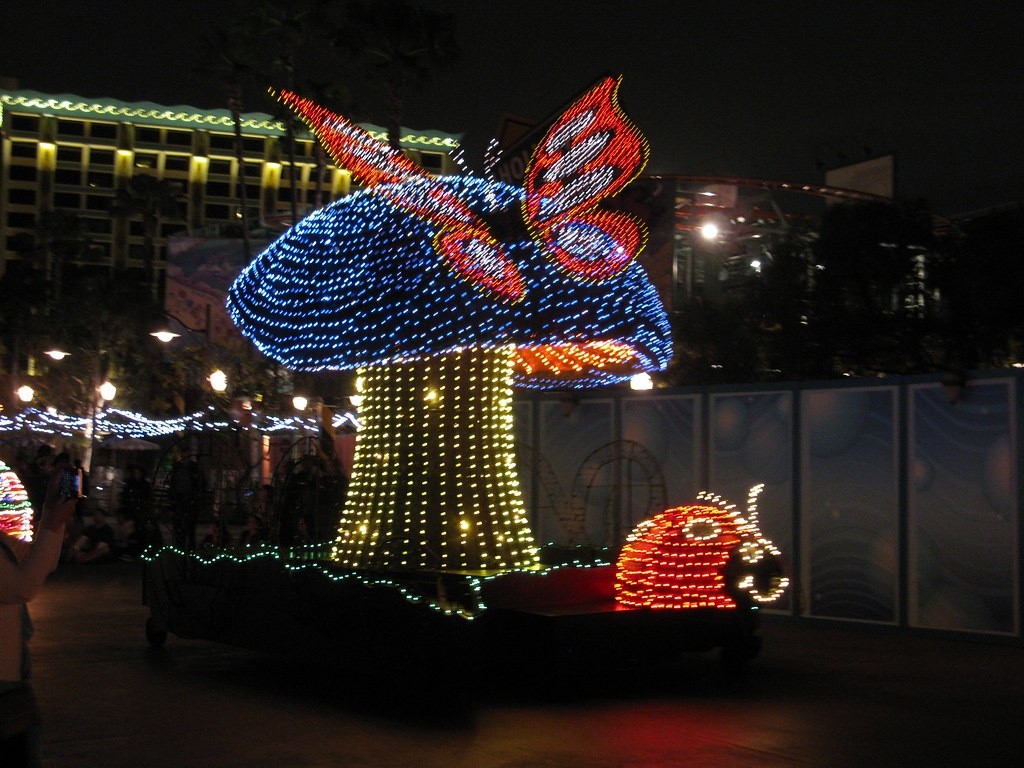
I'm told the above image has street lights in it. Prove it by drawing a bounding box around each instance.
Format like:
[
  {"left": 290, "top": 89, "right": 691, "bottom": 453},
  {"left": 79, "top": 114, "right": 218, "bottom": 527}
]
[{"left": 16, "top": 311, "right": 315, "bottom": 551}]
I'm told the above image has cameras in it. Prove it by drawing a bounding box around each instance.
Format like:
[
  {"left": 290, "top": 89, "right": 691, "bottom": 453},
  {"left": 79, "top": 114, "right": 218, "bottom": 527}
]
[{"left": 62, "top": 469, "right": 83, "bottom": 499}]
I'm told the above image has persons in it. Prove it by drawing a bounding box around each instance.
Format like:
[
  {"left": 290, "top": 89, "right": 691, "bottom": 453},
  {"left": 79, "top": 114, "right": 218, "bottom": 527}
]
[
  {"left": 0, "top": 466, "right": 79, "bottom": 768},
  {"left": 25, "top": 445, "right": 269, "bottom": 565}
]
